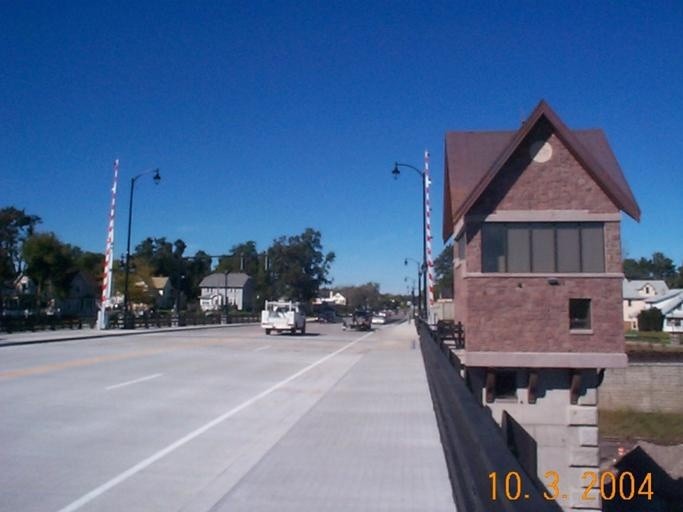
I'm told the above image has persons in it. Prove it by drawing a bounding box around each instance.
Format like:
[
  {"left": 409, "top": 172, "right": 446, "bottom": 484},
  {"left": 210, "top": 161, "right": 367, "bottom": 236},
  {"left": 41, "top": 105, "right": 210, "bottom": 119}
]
[
  {"left": 46, "top": 298, "right": 61, "bottom": 316},
  {"left": 171, "top": 304, "right": 179, "bottom": 328}
]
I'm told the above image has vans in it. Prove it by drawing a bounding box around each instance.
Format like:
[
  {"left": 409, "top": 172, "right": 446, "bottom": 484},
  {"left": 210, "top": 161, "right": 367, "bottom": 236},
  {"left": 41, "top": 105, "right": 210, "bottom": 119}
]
[{"left": 352, "top": 311, "right": 371, "bottom": 330}]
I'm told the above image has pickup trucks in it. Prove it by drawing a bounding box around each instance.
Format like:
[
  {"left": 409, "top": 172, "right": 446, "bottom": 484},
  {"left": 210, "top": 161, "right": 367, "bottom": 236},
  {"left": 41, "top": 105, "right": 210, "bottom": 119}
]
[{"left": 262, "top": 301, "right": 306, "bottom": 335}]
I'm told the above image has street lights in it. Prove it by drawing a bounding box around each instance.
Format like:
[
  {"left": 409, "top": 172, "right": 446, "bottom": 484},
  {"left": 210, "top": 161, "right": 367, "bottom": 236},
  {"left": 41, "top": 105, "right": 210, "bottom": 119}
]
[
  {"left": 392, "top": 162, "right": 427, "bottom": 320},
  {"left": 124, "top": 167, "right": 162, "bottom": 328},
  {"left": 223, "top": 269, "right": 232, "bottom": 316}
]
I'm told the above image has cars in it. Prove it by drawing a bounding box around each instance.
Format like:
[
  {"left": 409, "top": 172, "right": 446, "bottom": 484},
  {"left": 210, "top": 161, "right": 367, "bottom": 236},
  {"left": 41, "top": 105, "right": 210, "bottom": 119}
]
[
  {"left": 372, "top": 316, "right": 386, "bottom": 324},
  {"left": 379, "top": 311, "right": 391, "bottom": 317}
]
